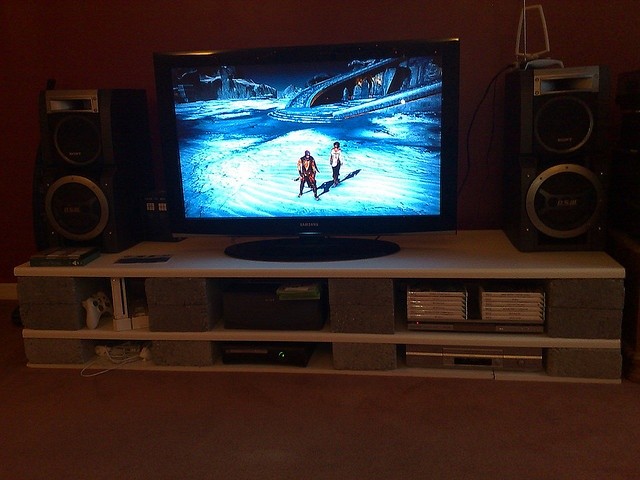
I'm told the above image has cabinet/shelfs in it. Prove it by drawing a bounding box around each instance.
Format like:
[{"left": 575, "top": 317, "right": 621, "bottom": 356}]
[{"left": 14, "top": 227, "right": 627, "bottom": 388}]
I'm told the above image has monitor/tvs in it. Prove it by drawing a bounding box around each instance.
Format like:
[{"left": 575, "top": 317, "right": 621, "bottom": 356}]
[{"left": 150, "top": 39, "right": 458, "bottom": 262}]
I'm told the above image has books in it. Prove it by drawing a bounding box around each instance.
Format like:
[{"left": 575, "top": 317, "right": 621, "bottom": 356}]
[
  {"left": 478, "top": 284, "right": 546, "bottom": 322},
  {"left": 406, "top": 281, "right": 468, "bottom": 320}
]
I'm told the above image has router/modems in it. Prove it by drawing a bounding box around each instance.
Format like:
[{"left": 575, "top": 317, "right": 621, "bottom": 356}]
[{"left": 519, "top": 1, "right": 565, "bottom": 69}]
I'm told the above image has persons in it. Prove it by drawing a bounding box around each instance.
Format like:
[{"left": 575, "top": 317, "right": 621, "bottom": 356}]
[
  {"left": 329, "top": 142, "right": 342, "bottom": 186},
  {"left": 294, "top": 150, "right": 320, "bottom": 201}
]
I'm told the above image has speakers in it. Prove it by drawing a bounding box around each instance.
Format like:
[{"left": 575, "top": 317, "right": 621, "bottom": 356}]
[
  {"left": 34, "top": 87, "right": 150, "bottom": 254},
  {"left": 496, "top": 61, "right": 615, "bottom": 254}
]
